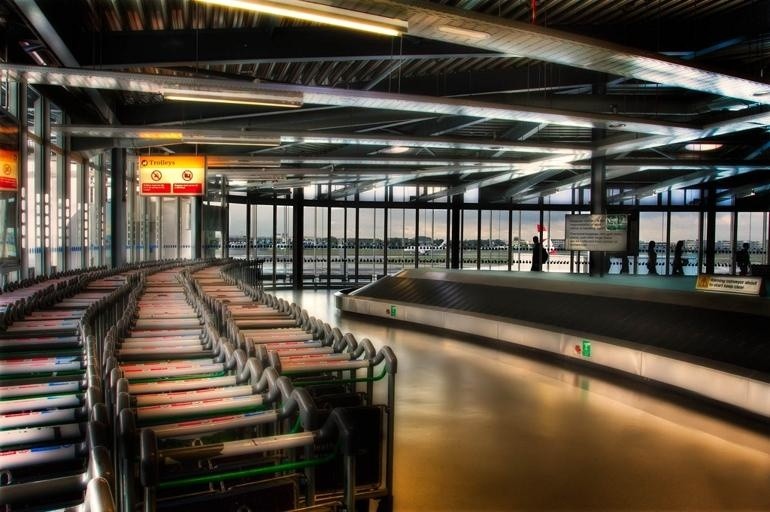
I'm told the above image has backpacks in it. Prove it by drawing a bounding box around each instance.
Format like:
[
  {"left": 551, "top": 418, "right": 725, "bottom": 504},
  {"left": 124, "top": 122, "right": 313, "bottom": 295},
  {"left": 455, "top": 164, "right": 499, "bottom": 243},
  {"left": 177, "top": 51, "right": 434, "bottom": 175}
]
[{"left": 541, "top": 247, "right": 549, "bottom": 264}]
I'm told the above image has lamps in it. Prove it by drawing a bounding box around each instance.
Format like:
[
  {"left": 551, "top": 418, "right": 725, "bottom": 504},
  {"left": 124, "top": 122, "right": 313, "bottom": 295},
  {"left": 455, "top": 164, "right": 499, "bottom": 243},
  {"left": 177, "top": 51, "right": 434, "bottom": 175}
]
[
  {"left": 160, "top": 89, "right": 303, "bottom": 109},
  {"left": 197, "top": 0, "right": 409, "bottom": 38}
]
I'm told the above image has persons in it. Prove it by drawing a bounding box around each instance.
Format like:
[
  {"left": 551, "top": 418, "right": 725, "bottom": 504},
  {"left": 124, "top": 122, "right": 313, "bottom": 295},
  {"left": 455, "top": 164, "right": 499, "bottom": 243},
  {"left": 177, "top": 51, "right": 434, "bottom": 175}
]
[
  {"left": 530, "top": 236, "right": 542, "bottom": 271},
  {"left": 647, "top": 241, "right": 658, "bottom": 275},
  {"left": 671, "top": 240, "right": 685, "bottom": 276},
  {"left": 736, "top": 243, "right": 751, "bottom": 279}
]
